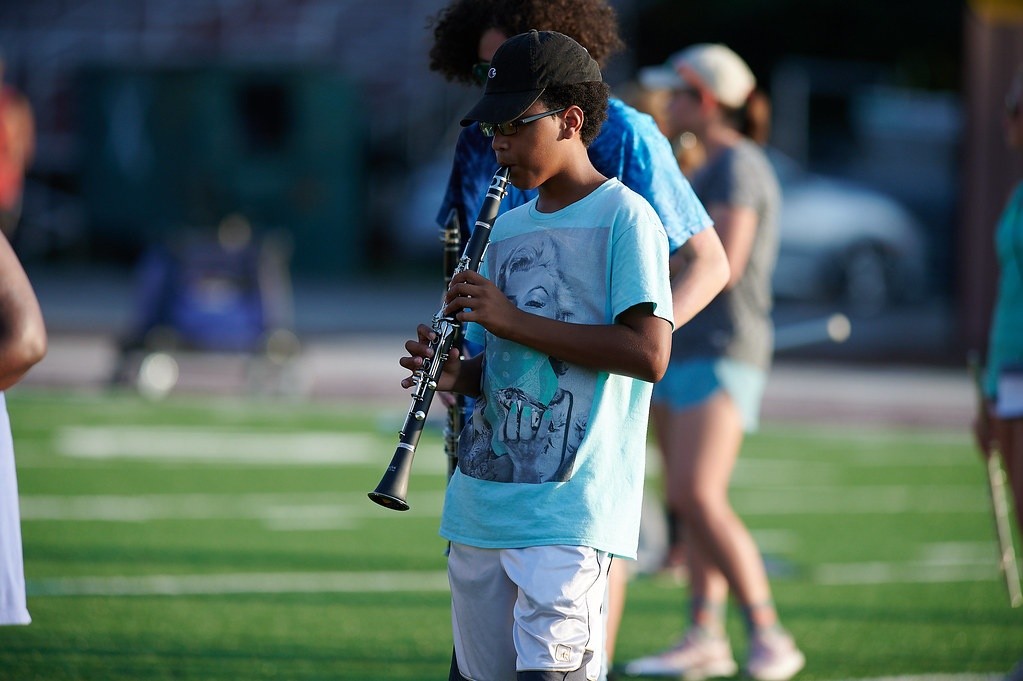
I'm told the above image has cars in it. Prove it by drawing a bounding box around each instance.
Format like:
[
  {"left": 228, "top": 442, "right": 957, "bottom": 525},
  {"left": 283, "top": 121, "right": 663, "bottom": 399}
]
[{"left": 388, "top": 148, "right": 938, "bottom": 308}]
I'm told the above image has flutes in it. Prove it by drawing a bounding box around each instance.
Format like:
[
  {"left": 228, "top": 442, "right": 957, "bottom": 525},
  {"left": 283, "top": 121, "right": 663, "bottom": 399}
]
[
  {"left": 437, "top": 210, "right": 462, "bottom": 559},
  {"left": 370, "top": 166, "right": 516, "bottom": 509}
]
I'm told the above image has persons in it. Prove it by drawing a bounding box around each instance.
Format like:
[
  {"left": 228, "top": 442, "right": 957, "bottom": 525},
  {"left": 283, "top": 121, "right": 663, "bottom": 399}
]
[
  {"left": 1, "top": 230, "right": 49, "bottom": 625},
  {"left": 973, "top": 184, "right": 1023, "bottom": 542},
  {"left": 98, "top": 216, "right": 261, "bottom": 391},
  {"left": 628, "top": 63, "right": 711, "bottom": 589},
  {"left": 626, "top": 43, "right": 805, "bottom": 681},
  {"left": 398, "top": 31, "right": 673, "bottom": 681},
  {"left": 428, "top": 0, "right": 731, "bottom": 680}
]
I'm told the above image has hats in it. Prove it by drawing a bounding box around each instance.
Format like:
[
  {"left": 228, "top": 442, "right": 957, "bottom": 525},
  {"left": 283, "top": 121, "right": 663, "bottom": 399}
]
[
  {"left": 637, "top": 42, "right": 757, "bottom": 110},
  {"left": 459, "top": 29, "right": 603, "bottom": 128}
]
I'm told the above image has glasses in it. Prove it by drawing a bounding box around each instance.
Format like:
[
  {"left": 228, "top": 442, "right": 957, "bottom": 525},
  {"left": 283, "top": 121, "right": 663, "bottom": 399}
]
[
  {"left": 472, "top": 61, "right": 493, "bottom": 81},
  {"left": 477, "top": 107, "right": 567, "bottom": 138}
]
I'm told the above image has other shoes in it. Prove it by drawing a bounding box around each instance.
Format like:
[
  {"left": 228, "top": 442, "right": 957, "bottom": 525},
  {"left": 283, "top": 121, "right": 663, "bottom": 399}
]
[
  {"left": 747, "top": 649, "right": 805, "bottom": 681},
  {"left": 623, "top": 645, "right": 738, "bottom": 680}
]
